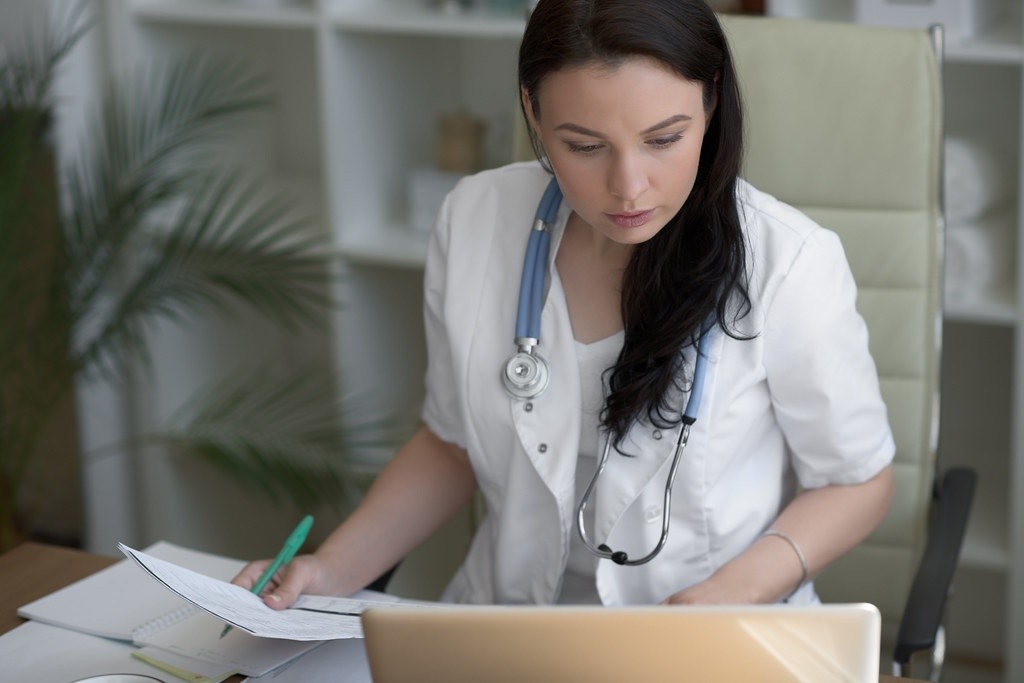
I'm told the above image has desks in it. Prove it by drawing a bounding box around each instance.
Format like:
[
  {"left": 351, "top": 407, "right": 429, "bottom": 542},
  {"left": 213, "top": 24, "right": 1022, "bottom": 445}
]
[{"left": 0, "top": 543, "right": 920, "bottom": 683}]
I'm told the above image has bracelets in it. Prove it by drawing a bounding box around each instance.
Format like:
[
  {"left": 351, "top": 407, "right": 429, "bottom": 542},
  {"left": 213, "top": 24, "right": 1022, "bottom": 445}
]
[{"left": 764, "top": 530, "right": 807, "bottom": 601}]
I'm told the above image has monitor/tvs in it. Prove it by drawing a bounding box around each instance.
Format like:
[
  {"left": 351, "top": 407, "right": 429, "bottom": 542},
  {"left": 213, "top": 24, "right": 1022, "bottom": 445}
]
[{"left": 359, "top": 601, "right": 884, "bottom": 683}]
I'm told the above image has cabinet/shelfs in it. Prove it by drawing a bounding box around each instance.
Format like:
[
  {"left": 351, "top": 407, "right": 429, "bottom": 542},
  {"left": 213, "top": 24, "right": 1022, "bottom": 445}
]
[{"left": 96, "top": 1, "right": 1024, "bottom": 683}]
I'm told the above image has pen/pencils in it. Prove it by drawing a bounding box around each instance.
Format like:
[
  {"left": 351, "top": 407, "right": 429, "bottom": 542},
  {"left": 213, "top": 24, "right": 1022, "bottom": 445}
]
[{"left": 215, "top": 513, "right": 317, "bottom": 640}]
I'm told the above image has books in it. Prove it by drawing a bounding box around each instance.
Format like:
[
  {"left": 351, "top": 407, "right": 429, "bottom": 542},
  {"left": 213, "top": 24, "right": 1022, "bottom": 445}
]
[{"left": 17, "top": 542, "right": 400, "bottom": 678}]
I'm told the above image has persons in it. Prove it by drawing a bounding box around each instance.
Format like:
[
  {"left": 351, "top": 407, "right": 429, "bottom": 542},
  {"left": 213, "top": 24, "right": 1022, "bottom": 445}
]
[{"left": 231, "top": 1, "right": 897, "bottom": 610}]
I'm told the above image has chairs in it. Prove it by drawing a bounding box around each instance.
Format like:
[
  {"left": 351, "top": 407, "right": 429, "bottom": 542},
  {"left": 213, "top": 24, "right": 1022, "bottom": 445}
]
[{"left": 506, "top": 13, "right": 976, "bottom": 683}]
